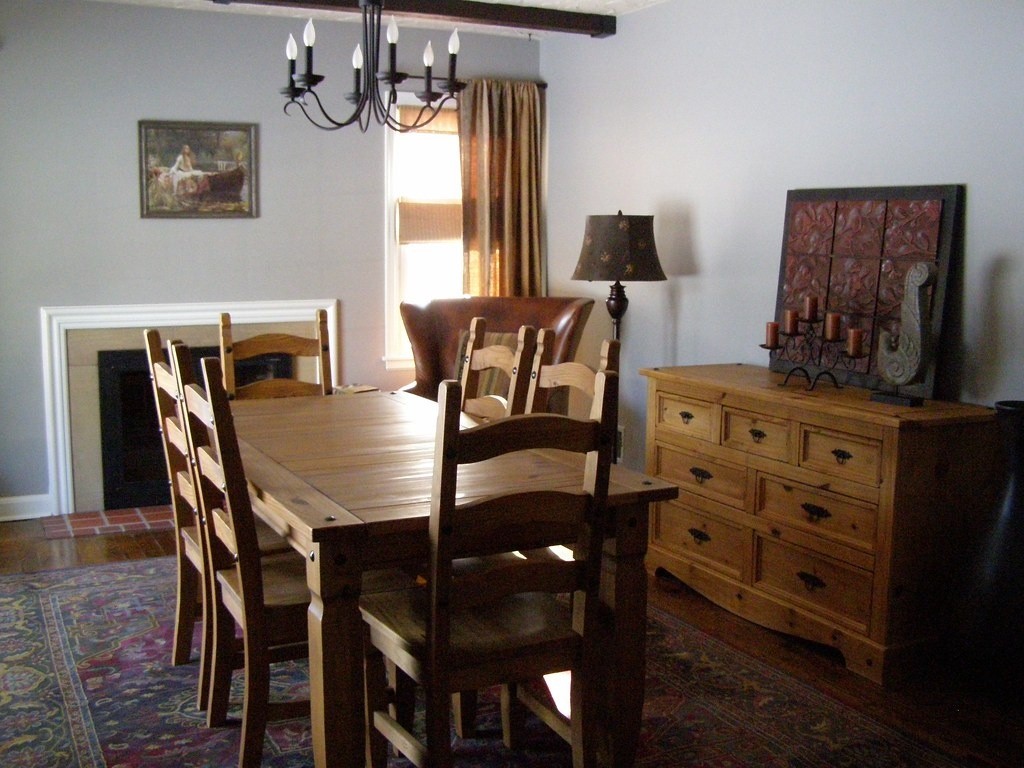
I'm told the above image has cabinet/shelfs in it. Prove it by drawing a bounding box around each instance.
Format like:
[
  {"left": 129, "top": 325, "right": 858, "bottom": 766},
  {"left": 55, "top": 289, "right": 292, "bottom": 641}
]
[{"left": 638, "top": 365, "right": 1007, "bottom": 696}]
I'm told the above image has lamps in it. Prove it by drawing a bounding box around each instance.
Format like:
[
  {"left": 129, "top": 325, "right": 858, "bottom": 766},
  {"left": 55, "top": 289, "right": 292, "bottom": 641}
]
[
  {"left": 279, "top": 0, "right": 468, "bottom": 133},
  {"left": 572, "top": 210, "right": 666, "bottom": 343}
]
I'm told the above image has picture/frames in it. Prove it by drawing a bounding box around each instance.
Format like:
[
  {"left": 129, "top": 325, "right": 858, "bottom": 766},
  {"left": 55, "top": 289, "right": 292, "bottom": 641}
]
[{"left": 138, "top": 119, "right": 259, "bottom": 219}]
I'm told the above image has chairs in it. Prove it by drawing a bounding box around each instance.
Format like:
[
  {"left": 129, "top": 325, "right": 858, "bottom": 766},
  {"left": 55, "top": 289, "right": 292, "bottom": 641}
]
[{"left": 143, "top": 294, "right": 618, "bottom": 768}]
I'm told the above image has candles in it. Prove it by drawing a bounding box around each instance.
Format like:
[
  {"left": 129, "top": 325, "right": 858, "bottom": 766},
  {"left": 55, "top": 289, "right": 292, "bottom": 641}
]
[
  {"left": 846, "top": 326, "right": 866, "bottom": 356},
  {"left": 784, "top": 308, "right": 799, "bottom": 333},
  {"left": 825, "top": 312, "right": 841, "bottom": 341},
  {"left": 767, "top": 322, "right": 780, "bottom": 347},
  {"left": 804, "top": 295, "right": 818, "bottom": 321}
]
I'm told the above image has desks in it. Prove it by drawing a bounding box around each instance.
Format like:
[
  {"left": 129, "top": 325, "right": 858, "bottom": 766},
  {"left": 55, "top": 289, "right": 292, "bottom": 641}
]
[{"left": 200, "top": 392, "right": 677, "bottom": 768}]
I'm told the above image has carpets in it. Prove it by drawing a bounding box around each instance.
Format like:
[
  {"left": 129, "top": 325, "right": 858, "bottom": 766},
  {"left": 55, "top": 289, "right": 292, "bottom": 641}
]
[{"left": 0, "top": 556, "right": 953, "bottom": 768}]
